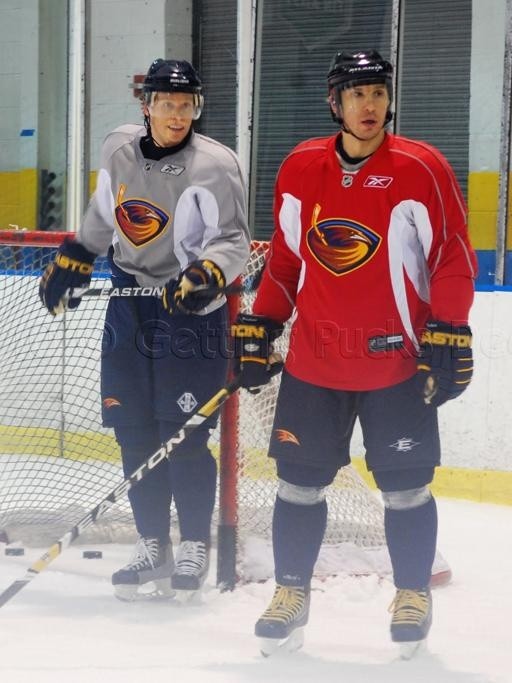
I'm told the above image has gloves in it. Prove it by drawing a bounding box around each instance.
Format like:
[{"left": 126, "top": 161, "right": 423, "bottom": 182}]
[
  {"left": 160, "top": 259, "right": 226, "bottom": 318},
  {"left": 38, "top": 236, "right": 99, "bottom": 317},
  {"left": 415, "top": 318, "right": 474, "bottom": 408},
  {"left": 230, "top": 311, "right": 286, "bottom": 389}
]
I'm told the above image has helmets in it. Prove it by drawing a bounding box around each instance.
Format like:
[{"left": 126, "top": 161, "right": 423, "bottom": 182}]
[
  {"left": 141, "top": 56, "right": 204, "bottom": 96},
  {"left": 327, "top": 48, "right": 395, "bottom": 87}
]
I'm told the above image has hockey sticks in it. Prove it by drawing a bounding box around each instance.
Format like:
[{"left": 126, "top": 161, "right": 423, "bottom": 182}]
[{"left": 0, "top": 345, "right": 274, "bottom": 609}]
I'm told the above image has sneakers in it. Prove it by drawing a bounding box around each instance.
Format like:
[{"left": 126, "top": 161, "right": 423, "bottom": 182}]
[
  {"left": 389, "top": 586, "right": 433, "bottom": 644},
  {"left": 254, "top": 583, "right": 311, "bottom": 640}
]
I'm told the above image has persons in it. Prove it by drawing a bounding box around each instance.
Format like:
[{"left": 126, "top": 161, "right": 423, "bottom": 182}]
[
  {"left": 39, "top": 58, "right": 251, "bottom": 591},
  {"left": 230, "top": 44, "right": 482, "bottom": 641}
]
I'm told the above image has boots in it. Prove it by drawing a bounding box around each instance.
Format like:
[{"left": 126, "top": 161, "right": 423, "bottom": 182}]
[
  {"left": 170, "top": 540, "right": 212, "bottom": 591},
  {"left": 110, "top": 533, "right": 176, "bottom": 587}
]
